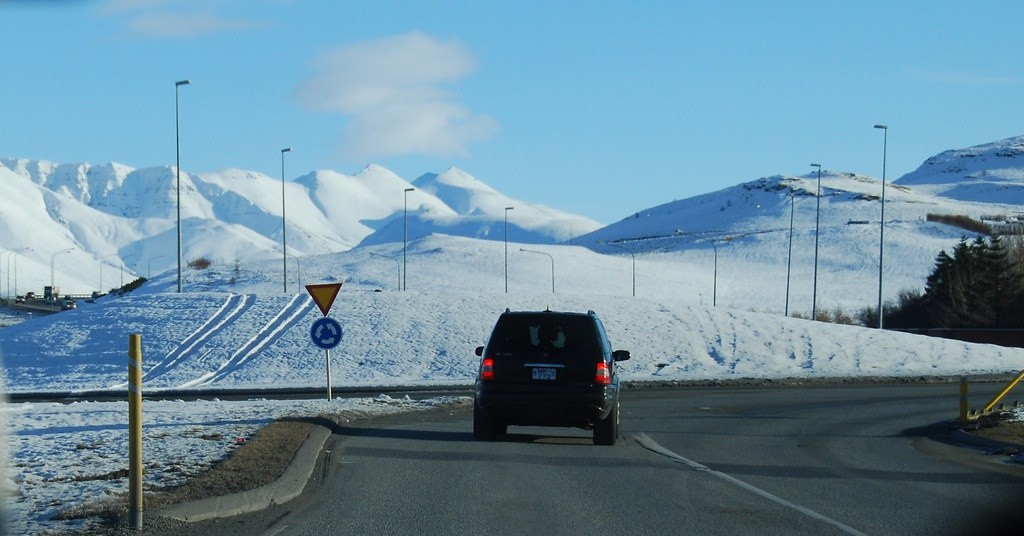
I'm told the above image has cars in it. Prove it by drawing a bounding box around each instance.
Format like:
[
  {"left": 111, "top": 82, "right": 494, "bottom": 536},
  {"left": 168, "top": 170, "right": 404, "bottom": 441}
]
[
  {"left": 15, "top": 295, "right": 26, "bottom": 304},
  {"left": 63, "top": 299, "right": 77, "bottom": 312},
  {"left": 26, "top": 291, "right": 36, "bottom": 299}
]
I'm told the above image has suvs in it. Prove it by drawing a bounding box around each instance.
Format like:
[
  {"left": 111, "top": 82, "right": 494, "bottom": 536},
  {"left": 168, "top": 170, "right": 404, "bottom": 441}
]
[{"left": 472, "top": 307, "right": 632, "bottom": 448}]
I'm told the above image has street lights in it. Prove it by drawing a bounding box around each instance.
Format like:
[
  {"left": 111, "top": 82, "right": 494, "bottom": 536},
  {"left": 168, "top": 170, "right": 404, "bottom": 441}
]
[
  {"left": 503, "top": 205, "right": 515, "bottom": 293},
  {"left": 50, "top": 247, "right": 75, "bottom": 299},
  {"left": 706, "top": 238, "right": 718, "bottom": 306},
  {"left": 121, "top": 253, "right": 143, "bottom": 297},
  {"left": 99, "top": 254, "right": 118, "bottom": 297},
  {"left": 369, "top": 252, "right": 400, "bottom": 291},
  {"left": 873, "top": 125, "right": 888, "bottom": 329},
  {"left": 607, "top": 242, "right": 636, "bottom": 298},
  {"left": 281, "top": 147, "right": 294, "bottom": 291},
  {"left": 147, "top": 255, "right": 164, "bottom": 280},
  {"left": 175, "top": 80, "right": 193, "bottom": 293},
  {"left": 284, "top": 253, "right": 301, "bottom": 293},
  {"left": 519, "top": 248, "right": 554, "bottom": 294},
  {"left": 7, "top": 247, "right": 29, "bottom": 302},
  {"left": 403, "top": 189, "right": 415, "bottom": 291},
  {"left": 810, "top": 163, "right": 821, "bottom": 319},
  {"left": 785, "top": 193, "right": 794, "bottom": 317}
]
